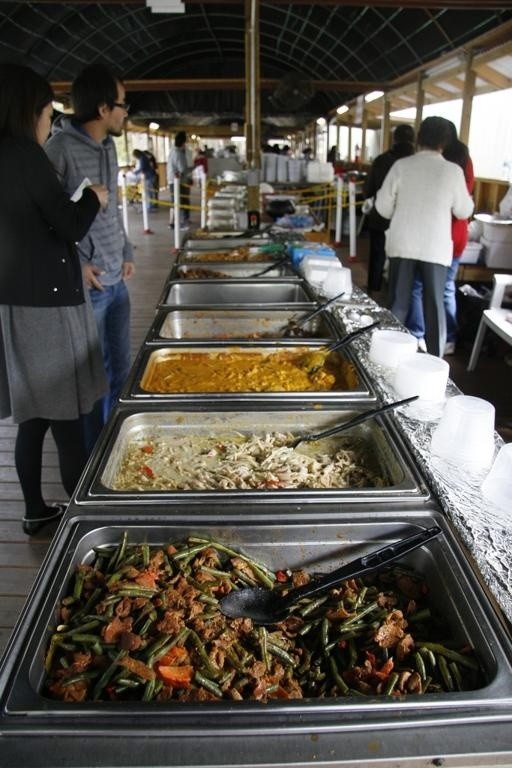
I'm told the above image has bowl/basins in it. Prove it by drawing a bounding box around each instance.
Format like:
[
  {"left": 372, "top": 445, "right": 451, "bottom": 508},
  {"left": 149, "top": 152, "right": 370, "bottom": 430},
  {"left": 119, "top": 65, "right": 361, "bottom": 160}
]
[
  {"left": 369, "top": 326, "right": 512, "bottom": 510},
  {"left": 324, "top": 266, "right": 353, "bottom": 294}
]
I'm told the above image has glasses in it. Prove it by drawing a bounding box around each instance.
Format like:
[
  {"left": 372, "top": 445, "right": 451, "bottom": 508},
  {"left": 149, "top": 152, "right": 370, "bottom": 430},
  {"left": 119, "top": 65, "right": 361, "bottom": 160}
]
[{"left": 116, "top": 103, "right": 131, "bottom": 112}]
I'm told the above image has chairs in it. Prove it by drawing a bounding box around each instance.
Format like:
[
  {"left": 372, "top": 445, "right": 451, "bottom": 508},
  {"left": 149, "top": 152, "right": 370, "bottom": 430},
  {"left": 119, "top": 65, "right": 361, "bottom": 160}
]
[{"left": 465, "top": 269, "right": 511, "bottom": 372}]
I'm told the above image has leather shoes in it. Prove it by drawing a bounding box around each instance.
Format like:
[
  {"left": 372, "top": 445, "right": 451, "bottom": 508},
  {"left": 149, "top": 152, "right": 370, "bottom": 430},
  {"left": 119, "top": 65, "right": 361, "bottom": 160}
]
[{"left": 22, "top": 503, "right": 71, "bottom": 536}]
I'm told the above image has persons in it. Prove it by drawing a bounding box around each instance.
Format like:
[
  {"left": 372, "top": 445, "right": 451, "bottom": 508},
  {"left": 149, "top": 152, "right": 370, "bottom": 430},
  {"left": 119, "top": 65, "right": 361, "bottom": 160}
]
[
  {"left": 132, "top": 148, "right": 156, "bottom": 211},
  {"left": 360, "top": 115, "right": 476, "bottom": 361},
  {"left": 166, "top": 129, "right": 241, "bottom": 230},
  {"left": 327, "top": 146, "right": 340, "bottom": 164},
  {"left": 144, "top": 150, "right": 160, "bottom": 209},
  {"left": 260, "top": 141, "right": 294, "bottom": 160},
  {"left": 303, "top": 147, "right": 312, "bottom": 166},
  {"left": 1, "top": 63, "right": 110, "bottom": 538},
  {"left": 41, "top": 67, "right": 136, "bottom": 426}
]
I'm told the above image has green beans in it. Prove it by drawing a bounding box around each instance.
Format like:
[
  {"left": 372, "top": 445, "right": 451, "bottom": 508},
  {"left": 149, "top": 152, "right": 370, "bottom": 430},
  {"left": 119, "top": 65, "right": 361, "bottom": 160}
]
[{"left": 44, "top": 531, "right": 484, "bottom": 700}]
